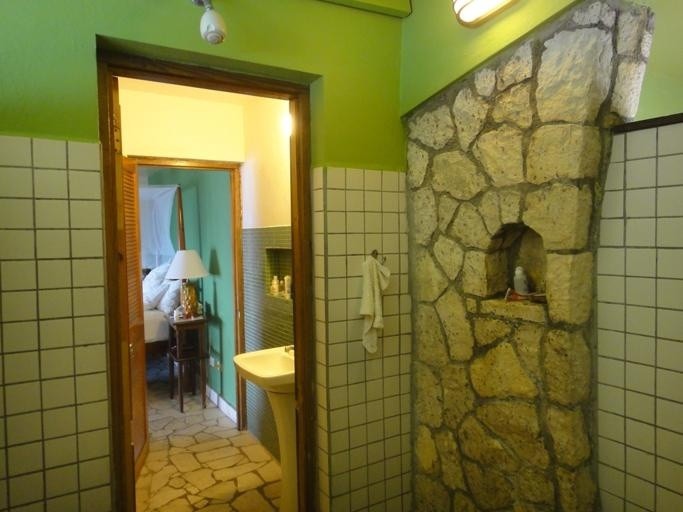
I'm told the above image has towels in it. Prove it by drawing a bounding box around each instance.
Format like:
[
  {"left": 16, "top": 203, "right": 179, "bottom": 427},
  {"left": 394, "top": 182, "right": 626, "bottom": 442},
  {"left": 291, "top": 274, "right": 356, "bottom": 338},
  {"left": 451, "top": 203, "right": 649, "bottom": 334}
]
[{"left": 358, "top": 254, "right": 392, "bottom": 355}]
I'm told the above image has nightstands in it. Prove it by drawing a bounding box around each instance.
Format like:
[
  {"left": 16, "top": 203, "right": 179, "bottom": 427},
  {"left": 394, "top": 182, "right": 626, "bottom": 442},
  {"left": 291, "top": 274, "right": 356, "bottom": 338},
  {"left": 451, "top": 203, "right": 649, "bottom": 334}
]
[{"left": 167, "top": 316, "right": 214, "bottom": 412}]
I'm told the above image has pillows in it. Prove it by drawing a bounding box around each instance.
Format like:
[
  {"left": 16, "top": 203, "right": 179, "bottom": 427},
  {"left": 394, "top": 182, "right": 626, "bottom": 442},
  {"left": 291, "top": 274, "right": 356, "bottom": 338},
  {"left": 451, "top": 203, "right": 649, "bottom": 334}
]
[
  {"left": 158, "top": 279, "right": 181, "bottom": 316},
  {"left": 143, "top": 262, "right": 167, "bottom": 310}
]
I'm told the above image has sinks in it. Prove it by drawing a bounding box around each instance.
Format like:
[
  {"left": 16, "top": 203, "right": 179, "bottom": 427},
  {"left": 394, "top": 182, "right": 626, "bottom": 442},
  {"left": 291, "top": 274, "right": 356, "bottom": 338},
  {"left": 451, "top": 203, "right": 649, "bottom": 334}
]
[{"left": 233, "top": 345, "right": 295, "bottom": 394}]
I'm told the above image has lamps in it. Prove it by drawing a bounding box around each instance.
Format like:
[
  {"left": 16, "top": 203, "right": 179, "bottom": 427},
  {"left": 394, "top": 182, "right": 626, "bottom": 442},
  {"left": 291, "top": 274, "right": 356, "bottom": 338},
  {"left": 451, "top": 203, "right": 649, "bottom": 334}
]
[
  {"left": 281, "top": 111, "right": 297, "bottom": 136},
  {"left": 450, "top": 0, "right": 530, "bottom": 24},
  {"left": 165, "top": 249, "right": 207, "bottom": 317}
]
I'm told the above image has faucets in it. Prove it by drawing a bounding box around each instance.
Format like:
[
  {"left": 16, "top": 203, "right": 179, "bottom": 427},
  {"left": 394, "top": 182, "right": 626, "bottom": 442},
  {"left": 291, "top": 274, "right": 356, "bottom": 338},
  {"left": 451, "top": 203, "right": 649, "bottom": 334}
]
[{"left": 285, "top": 346, "right": 294, "bottom": 353}]
